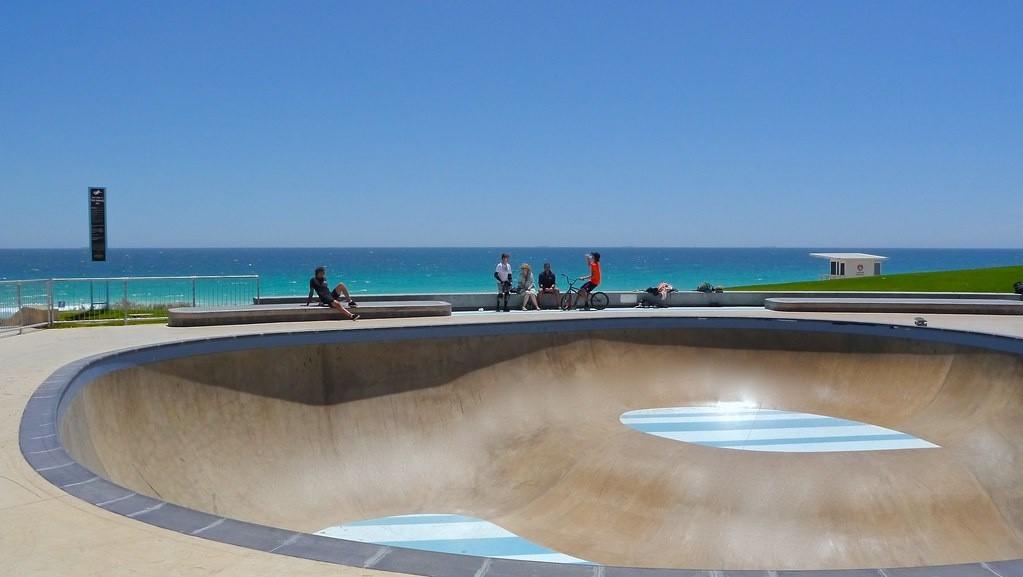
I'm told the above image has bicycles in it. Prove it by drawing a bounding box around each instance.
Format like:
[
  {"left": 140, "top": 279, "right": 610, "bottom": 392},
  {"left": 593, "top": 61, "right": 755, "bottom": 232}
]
[{"left": 560, "top": 274, "right": 609, "bottom": 311}]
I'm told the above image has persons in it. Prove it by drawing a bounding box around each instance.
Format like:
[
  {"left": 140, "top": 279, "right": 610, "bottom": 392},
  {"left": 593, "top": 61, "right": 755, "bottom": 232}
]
[
  {"left": 538, "top": 263, "right": 561, "bottom": 309},
  {"left": 569, "top": 253, "right": 601, "bottom": 311},
  {"left": 301, "top": 267, "right": 360, "bottom": 321},
  {"left": 1014, "top": 282, "right": 1023, "bottom": 300},
  {"left": 519, "top": 263, "right": 540, "bottom": 311},
  {"left": 494, "top": 254, "right": 513, "bottom": 312}
]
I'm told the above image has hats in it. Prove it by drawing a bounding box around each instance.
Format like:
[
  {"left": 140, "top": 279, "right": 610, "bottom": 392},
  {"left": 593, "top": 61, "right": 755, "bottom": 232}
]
[
  {"left": 502, "top": 254, "right": 510, "bottom": 257},
  {"left": 591, "top": 251, "right": 600, "bottom": 262},
  {"left": 521, "top": 263, "right": 529, "bottom": 270}
]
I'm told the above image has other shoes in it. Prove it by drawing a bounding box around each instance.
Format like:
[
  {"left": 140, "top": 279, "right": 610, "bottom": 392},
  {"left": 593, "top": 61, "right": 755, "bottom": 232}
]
[
  {"left": 570, "top": 305, "right": 576, "bottom": 309},
  {"left": 580, "top": 306, "right": 590, "bottom": 311},
  {"left": 352, "top": 314, "right": 360, "bottom": 320},
  {"left": 537, "top": 306, "right": 540, "bottom": 310},
  {"left": 349, "top": 301, "right": 358, "bottom": 307},
  {"left": 522, "top": 307, "right": 527, "bottom": 310}
]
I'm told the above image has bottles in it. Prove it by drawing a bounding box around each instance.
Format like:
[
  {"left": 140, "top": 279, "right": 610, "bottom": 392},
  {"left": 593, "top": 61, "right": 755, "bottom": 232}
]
[{"left": 585, "top": 253, "right": 592, "bottom": 259}]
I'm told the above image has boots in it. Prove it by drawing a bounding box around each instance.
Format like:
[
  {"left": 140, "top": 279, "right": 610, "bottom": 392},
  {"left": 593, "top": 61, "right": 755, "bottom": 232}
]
[
  {"left": 503, "top": 300, "right": 510, "bottom": 312},
  {"left": 496, "top": 301, "right": 500, "bottom": 312}
]
